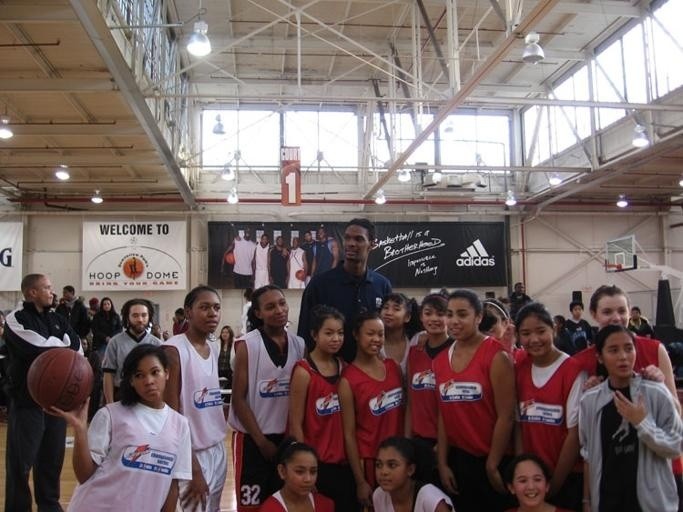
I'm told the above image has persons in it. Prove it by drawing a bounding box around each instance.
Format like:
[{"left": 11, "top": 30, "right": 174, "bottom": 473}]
[
  {"left": 229, "top": 283, "right": 683, "bottom": 511},
  {"left": 219, "top": 224, "right": 339, "bottom": 290},
  {"left": 1, "top": 273, "right": 237, "bottom": 511},
  {"left": 297, "top": 218, "right": 393, "bottom": 361}
]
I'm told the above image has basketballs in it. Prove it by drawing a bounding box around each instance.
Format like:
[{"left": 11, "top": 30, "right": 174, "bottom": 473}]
[
  {"left": 295, "top": 270, "right": 304, "bottom": 278},
  {"left": 225, "top": 252, "right": 235, "bottom": 264},
  {"left": 28, "top": 348, "right": 94, "bottom": 413}
]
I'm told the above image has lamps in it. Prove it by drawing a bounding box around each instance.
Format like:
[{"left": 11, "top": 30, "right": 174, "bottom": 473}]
[
  {"left": 368, "top": 1, "right": 546, "bottom": 205},
  {"left": 0, "top": 117, "right": 106, "bottom": 207},
  {"left": 502, "top": 106, "right": 650, "bottom": 209},
  {"left": 106, "top": 1, "right": 240, "bottom": 206}
]
